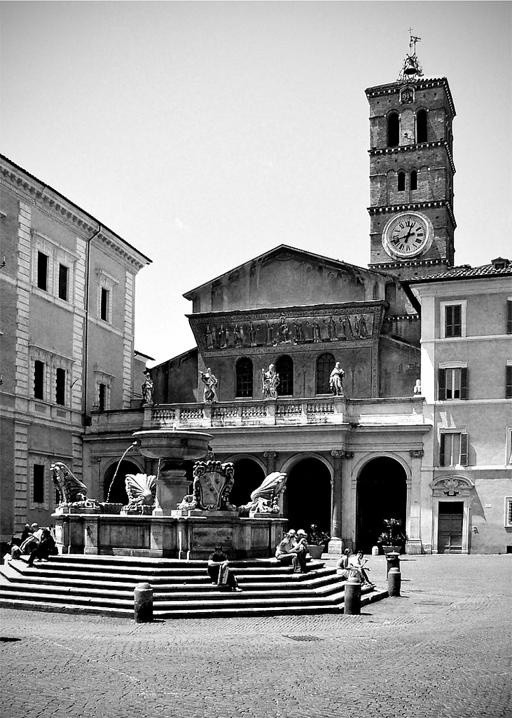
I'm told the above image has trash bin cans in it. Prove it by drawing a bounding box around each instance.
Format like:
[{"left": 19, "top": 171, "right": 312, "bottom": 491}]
[{"left": 386, "top": 552, "right": 400, "bottom": 581}]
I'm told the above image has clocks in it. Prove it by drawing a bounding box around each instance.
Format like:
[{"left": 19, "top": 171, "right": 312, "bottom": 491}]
[{"left": 381, "top": 210, "right": 434, "bottom": 262}]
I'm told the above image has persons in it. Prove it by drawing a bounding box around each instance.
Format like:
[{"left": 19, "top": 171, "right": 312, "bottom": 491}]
[
  {"left": 262, "top": 364, "right": 280, "bottom": 398},
  {"left": 200, "top": 367, "right": 219, "bottom": 402},
  {"left": 19, "top": 523, "right": 58, "bottom": 568},
  {"left": 142, "top": 373, "right": 154, "bottom": 404},
  {"left": 275, "top": 529, "right": 309, "bottom": 573},
  {"left": 207, "top": 546, "right": 243, "bottom": 592},
  {"left": 336, "top": 548, "right": 375, "bottom": 586},
  {"left": 329, "top": 362, "right": 346, "bottom": 395}
]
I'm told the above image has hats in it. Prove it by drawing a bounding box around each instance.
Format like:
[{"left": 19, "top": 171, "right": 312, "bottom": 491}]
[{"left": 286, "top": 528, "right": 308, "bottom": 538}]
